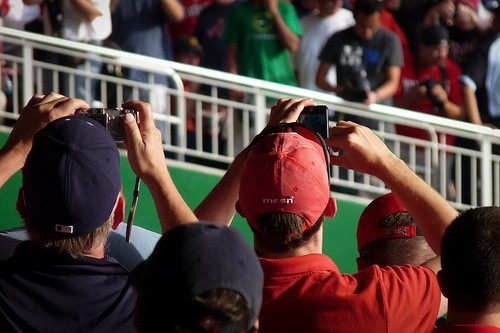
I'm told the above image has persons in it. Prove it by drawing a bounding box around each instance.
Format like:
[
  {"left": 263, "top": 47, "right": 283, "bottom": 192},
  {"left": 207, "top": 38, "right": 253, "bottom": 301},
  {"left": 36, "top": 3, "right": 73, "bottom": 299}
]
[
  {"left": 0, "top": 91, "right": 199, "bottom": 333},
  {"left": 356, "top": 191, "right": 437, "bottom": 273},
  {"left": 293, "top": 0, "right": 356, "bottom": 90},
  {"left": 0, "top": 0, "right": 233, "bottom": 158},
  {"left": 380, "top": 0, "right": 500, "bottom": 206},
  {"left": 194, "top": 96, "right": 461, "bottom": 333},
  {"left": 433, "top": 206, "right": 500, "bottom": 333},
  {"left": 222, "top": 0, "right": 304, "bottom": 158},
  {"left": 129, "top": 224, "right": 263, "bottom": 333},
  {"left": 315, "top": 0, "right": 402, "bottom": 199}
]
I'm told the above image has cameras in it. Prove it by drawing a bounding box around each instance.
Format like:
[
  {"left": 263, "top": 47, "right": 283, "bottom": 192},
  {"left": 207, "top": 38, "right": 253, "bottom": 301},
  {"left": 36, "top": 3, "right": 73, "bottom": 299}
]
[
  {"left": 423, "top": 79, "right": 445, "bottom": 111},
  {"left": 339, "top": 86, "right": 368, "bottom": 102},
  {"left": 271, "top": 105, "right": 330, "bottom": 140},
  {"left": 75, "top": 107, "right": 137, "bottom": 141}
]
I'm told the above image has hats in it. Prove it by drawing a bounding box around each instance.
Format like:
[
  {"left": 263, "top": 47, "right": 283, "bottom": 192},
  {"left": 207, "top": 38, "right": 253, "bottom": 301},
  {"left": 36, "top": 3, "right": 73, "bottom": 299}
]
[
  {"left": 417, "top": 25, "right": 449, "bottom": 45},
  {"left": 356, "top": 192, "right": 422, "bottom": 251},
  {"left": 128, "top": 222, "right": 264, "bottom": 331},
  {"left": 20, "top": 115, "right": 122, "bottom": 240},
  {"left": 239, "top": 124, "right": 330, "bottom": 240}
]
